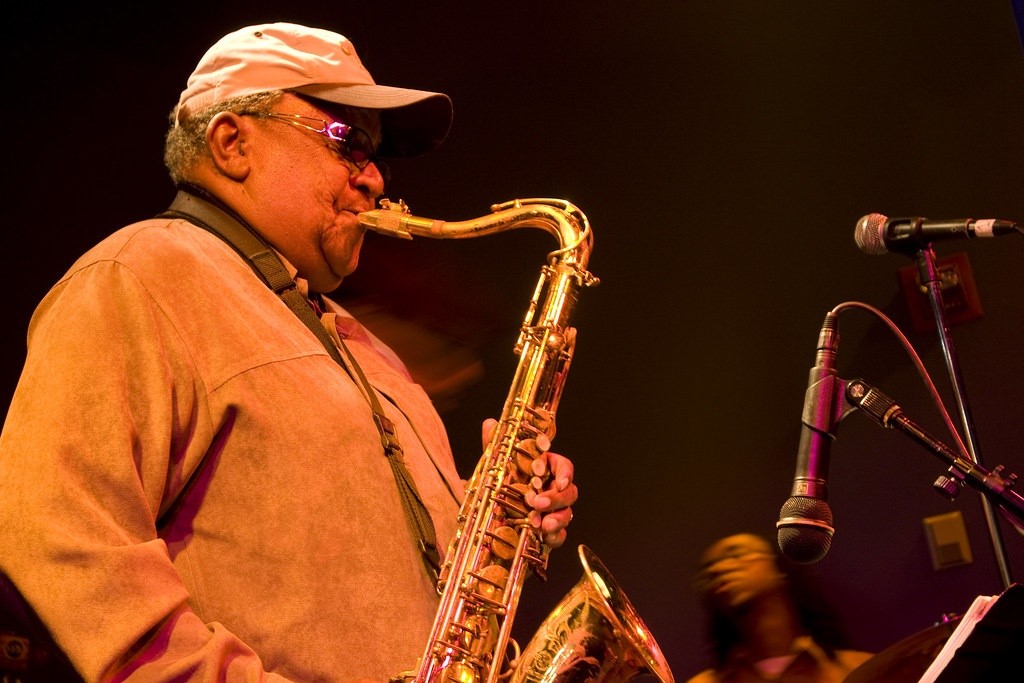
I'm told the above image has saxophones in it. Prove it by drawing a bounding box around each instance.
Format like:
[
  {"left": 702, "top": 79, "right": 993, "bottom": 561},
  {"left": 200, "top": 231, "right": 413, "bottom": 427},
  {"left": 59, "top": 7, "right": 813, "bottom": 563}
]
[{"left": 358, "top": 197, "right": 675, "bottom": 683}]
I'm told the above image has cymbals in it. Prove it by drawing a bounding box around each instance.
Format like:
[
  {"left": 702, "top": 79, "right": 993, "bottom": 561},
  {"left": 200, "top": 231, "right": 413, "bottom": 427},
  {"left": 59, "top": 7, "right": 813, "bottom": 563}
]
[{"left": 842, "top": 611, "right": 966, "bottom": 683}]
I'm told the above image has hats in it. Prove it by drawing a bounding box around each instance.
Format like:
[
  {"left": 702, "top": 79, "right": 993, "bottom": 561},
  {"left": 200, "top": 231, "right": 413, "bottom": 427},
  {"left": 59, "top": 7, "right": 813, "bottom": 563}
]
[{"left": 174, "top": 21, "right": 454, "bottom": 160}]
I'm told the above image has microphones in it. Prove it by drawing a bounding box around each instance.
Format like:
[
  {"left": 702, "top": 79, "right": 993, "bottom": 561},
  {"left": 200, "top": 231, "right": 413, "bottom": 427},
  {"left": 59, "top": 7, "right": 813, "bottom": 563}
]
[
  {"left": 854, "top": 213, "right": 1016, "bottom": 256},
  {"left": 776, "top": 311, "right": 842, "bottom": 563}
]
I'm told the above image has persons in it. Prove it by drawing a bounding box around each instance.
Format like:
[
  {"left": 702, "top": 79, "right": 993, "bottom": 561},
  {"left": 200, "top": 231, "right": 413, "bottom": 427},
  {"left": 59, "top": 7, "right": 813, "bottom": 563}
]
[
  {"left": 0, "top": 17, "right": 578, "bottom": 683},
  {"left": 685, "top": 529, "right": 877, "bottom": 683}
]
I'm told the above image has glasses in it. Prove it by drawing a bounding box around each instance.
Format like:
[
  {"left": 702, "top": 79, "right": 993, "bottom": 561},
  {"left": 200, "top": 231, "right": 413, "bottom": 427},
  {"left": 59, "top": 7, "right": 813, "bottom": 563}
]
[
  {"left": 701, "top": 547, "right": 779, "bottom": 573},
  {"left": 266, "top": 110, "right": 374, "bottom": 171}
]
